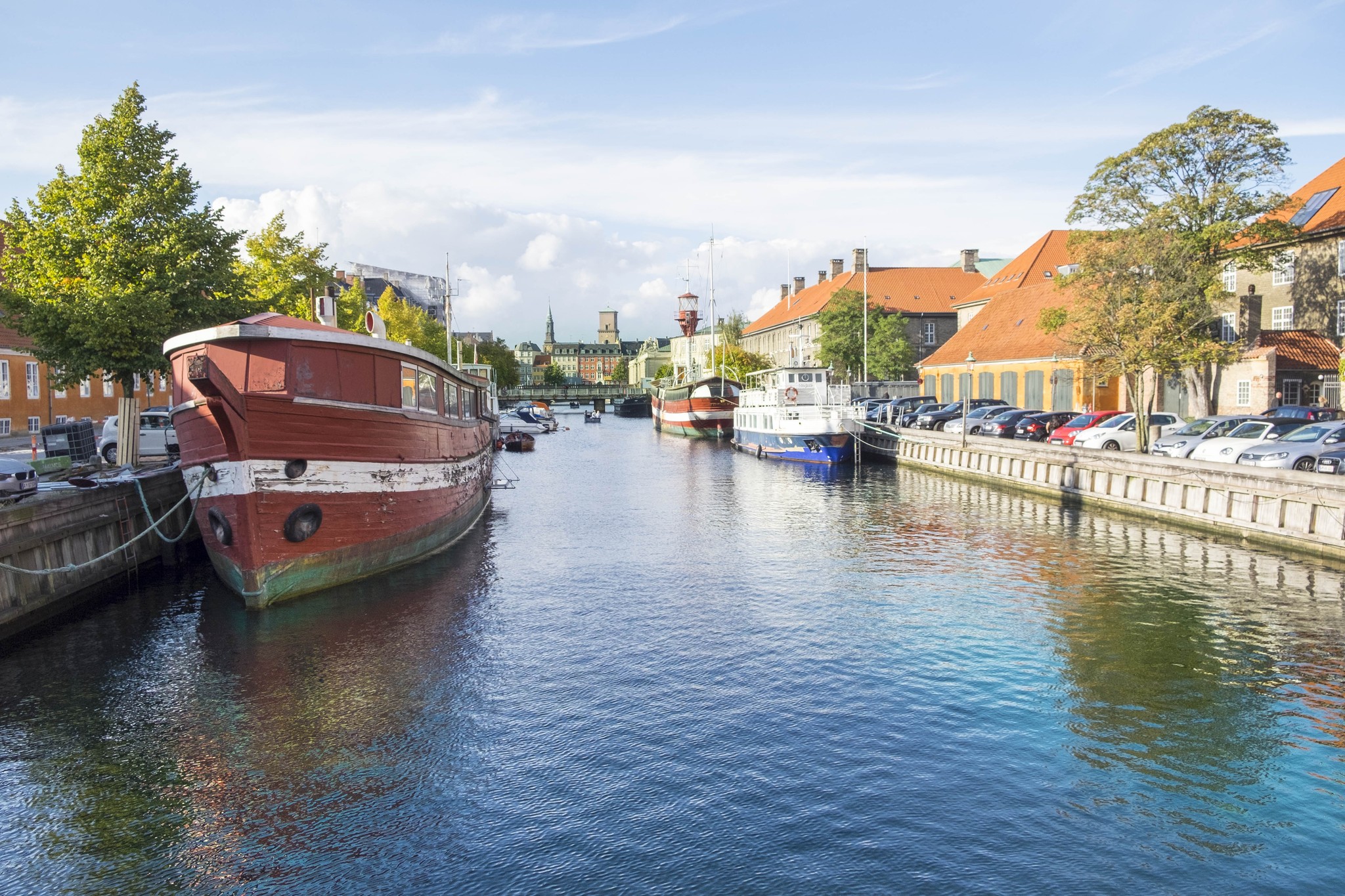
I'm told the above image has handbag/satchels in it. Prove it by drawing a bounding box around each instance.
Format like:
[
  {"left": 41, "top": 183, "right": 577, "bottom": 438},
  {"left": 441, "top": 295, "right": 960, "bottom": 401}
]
[{"left": 600, "top": 416, "right": 602, "bottom": 418}]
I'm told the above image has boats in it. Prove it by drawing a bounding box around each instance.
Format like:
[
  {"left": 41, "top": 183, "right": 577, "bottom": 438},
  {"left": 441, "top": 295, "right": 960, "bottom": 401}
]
[
  {"left": 584, "top": 414, "right": 601, "bottom": 422},
  {"left": 730, "top": 315, "right": 867, "bottom": 465},
  {"left": 569, "top": 405, "right": 580, "bottom": 408},
  {"left": 499, "top": 400, "right": 559, "bottom": 434},
  {"left": 613, "top": 392, "right": 652, "bottom": 418},
  {"left": 161, "top": 251, "right": 520, "bottom": 612},
  {"left": 503, "top": 429, "right": 536, "bottom": 452}
]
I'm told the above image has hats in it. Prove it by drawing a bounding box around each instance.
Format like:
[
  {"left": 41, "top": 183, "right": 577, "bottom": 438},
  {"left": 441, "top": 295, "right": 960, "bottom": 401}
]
[{"left": 597, "top": 411, "right": 600, "bottom": 413}]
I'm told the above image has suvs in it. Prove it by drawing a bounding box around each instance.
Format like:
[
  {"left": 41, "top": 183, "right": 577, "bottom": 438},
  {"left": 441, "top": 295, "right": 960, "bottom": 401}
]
[{"left": 1013, "top": 410, "right": 1082, "bottom": 443}]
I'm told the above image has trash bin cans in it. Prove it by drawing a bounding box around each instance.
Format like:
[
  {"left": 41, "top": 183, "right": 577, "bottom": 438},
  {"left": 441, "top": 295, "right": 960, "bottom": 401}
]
[{"left": 1147, "top": 425, "right": 1161, "bottom": 453}]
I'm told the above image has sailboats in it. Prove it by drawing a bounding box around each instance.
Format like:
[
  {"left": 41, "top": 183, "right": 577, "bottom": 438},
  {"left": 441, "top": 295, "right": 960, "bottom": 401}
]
[{"left": 648, "top": 224, "right": 750, "bottom": 439}]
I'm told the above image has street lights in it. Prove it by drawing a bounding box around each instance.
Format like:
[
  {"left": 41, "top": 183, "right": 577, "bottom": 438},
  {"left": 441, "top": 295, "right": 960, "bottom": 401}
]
[
  {"left": 1051, "top": 352, "right": 1059, "bottom": 412},
  {"left": 828, "top": 360, "right": 835, "bottom": 388},
  {"left": 846, "top": 365, "right": 852, "bottom": 385},
  {"left": 965, "top": 350, "right": 976, "bottom": 414}
]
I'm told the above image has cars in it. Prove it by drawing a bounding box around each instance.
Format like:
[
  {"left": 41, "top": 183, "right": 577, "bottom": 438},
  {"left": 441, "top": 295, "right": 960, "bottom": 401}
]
[
  {"left": 1048, "top": 410, "right": 1130, "bottom": 445},
  {"left": 1260, "top": 406, "right": 1345, "bottom": 423},
  {"left": 1313, "top": 448, "right": 1345, "bottom": 476},
  {"left": 0, "top": 456, "right": 39, "bottom": 505},
  {"left": 99, "top": 411, "right": 178, "bottom": 464},
  {"left": 1073, "top": 412, "right": 1188, "bottom": 451},
  {"left": 143, "top": 405, "right": 168, "bottom": 412},
  {"left": 1189, "top": 417, "right": 1317, "bottom": 464},
  {"left": 1238, "top": 419, "right": 1345, "bottom": 472},
  {"left": 851, "top": 395, "right": 1046, "bottom": 440},
  {"left": 1150, "top": 415, "right": 1268, "bottom": 458}
]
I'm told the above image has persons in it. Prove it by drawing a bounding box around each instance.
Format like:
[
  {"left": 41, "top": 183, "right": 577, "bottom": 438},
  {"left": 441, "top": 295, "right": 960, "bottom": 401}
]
[
  {"left": 1318, "top": 395, "right": 1329, "bottom": 419},
  {"left": 534, "top": 382, "right": 541, "bottom": 389},
  {"left": 1046, "top": 415, "right": 1062, "bottom": 435},
  {"left": 588, "top": 410, "right": 601, "bottom": 419},
  {"left": 563, "top": 385, "right": 568, "bottom": 394},
  {"left": 1269, "top": 392, "right": 1282, "bottom": 413},
  {"left": 576, "top": 401, "right": 579, "bottom": 406},
  {"left": 584, "top": 410, "right": 587, "bottom": 416},
  {"left": 570, "top": 402, "right": 575, "bottom": 406},
  {"left": 881, "top": 390, "right": 889, "bottom": 399}
]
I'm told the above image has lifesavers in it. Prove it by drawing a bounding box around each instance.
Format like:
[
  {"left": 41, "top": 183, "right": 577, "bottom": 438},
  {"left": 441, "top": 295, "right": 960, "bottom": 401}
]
[{"left": 784, "top": 387, "right": 798, "bottom": 401}]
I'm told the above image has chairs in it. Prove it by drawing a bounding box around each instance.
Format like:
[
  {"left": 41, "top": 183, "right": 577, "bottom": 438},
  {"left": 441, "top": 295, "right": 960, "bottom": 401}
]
[
  {"left": 165, "top": 420, "right": 170, "bottom": 426},
  {"left": 1225, "top": 421, "right": 1235, "bottom": 436},
  {"left": 1214, "top": 422, "right": 1224, "bottom": 436},
  {"left": 140, "top": 418, "right": 146, "bottom": 426}
]
